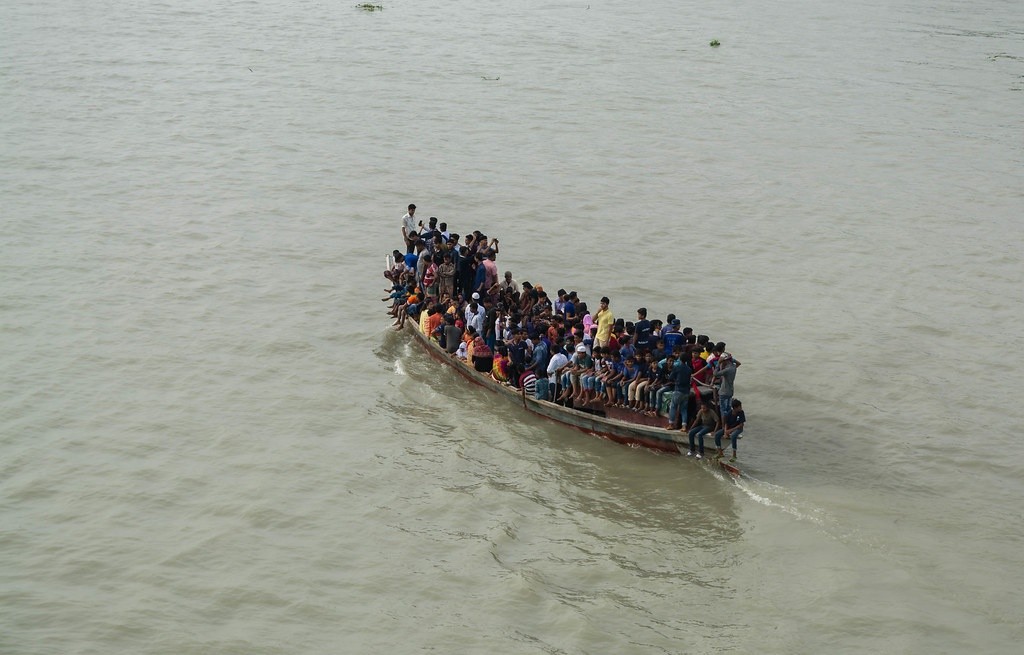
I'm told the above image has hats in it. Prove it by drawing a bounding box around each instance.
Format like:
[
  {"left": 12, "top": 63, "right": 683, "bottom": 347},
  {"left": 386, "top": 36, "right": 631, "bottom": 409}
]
[
  {"left": 669, "top": 319, "right": 680, "bottom": 327},
  {"left": 577, "top": 346, "right": 586, "bottom": 352},
  {"left": 509, "top": 313, "right": 521, "bottom": 322},
  {"left": 472, "top": 293, "right": 480, "bottom": 299}
]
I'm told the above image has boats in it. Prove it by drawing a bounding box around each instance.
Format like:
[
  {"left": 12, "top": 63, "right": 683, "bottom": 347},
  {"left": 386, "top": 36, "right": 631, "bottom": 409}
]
[{"left": 406, "top": 315, "right": 743, "bottom": 459}]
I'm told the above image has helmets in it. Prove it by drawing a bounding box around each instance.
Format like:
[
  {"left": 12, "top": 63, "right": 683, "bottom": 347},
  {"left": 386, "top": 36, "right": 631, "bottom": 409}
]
[{"left": 720, "top": 352, "right": 732, "bottom": 361}]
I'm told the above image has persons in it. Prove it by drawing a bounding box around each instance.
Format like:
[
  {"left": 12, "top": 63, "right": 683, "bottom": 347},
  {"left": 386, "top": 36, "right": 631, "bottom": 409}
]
[
  {"left": 419, "top": 298, "right": 433, "bottom": 336},
  {"left": 634, "top": 307, "right": 650, "bottom": 352},
  {"left": 546, "top": 344, "right": 568, "bottom": 403},
  {"left": 626, "top": 351, "right": 654, "bottom": 410},
  {"left": 714, "top": 399, "right": 747, "bottom": 462},
  {"left": 402, "top": 204, "right": 417, "bottom": 254},
  {"left": 644, "top": 356, "right": 676, "bottom": 418},
  {"left": 470, "top": 302, "right": 482, "bottom": 339},
  {"left": 635, "top": 359, "right": 665, "bottom": 414},
  {"left": 471, "top": 252, "right": 487, "bottom": 293},
  {"left": 437, "top": 253, "right": 455, "bottom": 299},
  {"left": 443, "top": 317, "right": 463, "bottom": 354},
  {"left": 591, "top": 296, "right": 614, "bottom": 350},
  {"left": 713, "top": 352, "right": 742, "bottom": 428},
  {"left": 382, "top": 217, "right": 741, "bottom": 436},
  {"left": 667, "top": 352, "right": 692, "bottom": 431},
  {"left": 492, "top": 345, "right": 516, "bottom": 387},
  {"left": 465, "top": 293, "right": 485, "bottom": 334},
  {"left": 525, "top": 330, "right": 549, "bottom": 378},
  {"left": 498, "top": 271, "right": 518, "bottom": 297},
  {"left": 687, "top": 402, "right": 719, "bottom": 459}
]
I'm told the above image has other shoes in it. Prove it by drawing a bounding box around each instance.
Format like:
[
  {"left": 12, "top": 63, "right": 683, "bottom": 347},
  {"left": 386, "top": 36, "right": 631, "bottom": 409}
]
[
  {"left": 381, "top": 289, "right": 404, "bottom": 332},
  {"left": 556, "top": 386, "right": 658, "bottom": 418},
  {"left": 713, "top": 453, "right": 725, "bottom": 459},
  {"left": 680, "top": 426, "right": 686, "bottom": 431},
  {"left": 729, "top": 457, "right": 737, "bottom": 462},
  {"left": 687, "top": 451, "right": 696, "bottom": 456},
  {"left": 667, "top": 424, "right": 673, "bottom": 430},
  {"left": 695, "top": 453, "right": 704, "bottom": 460}
]
